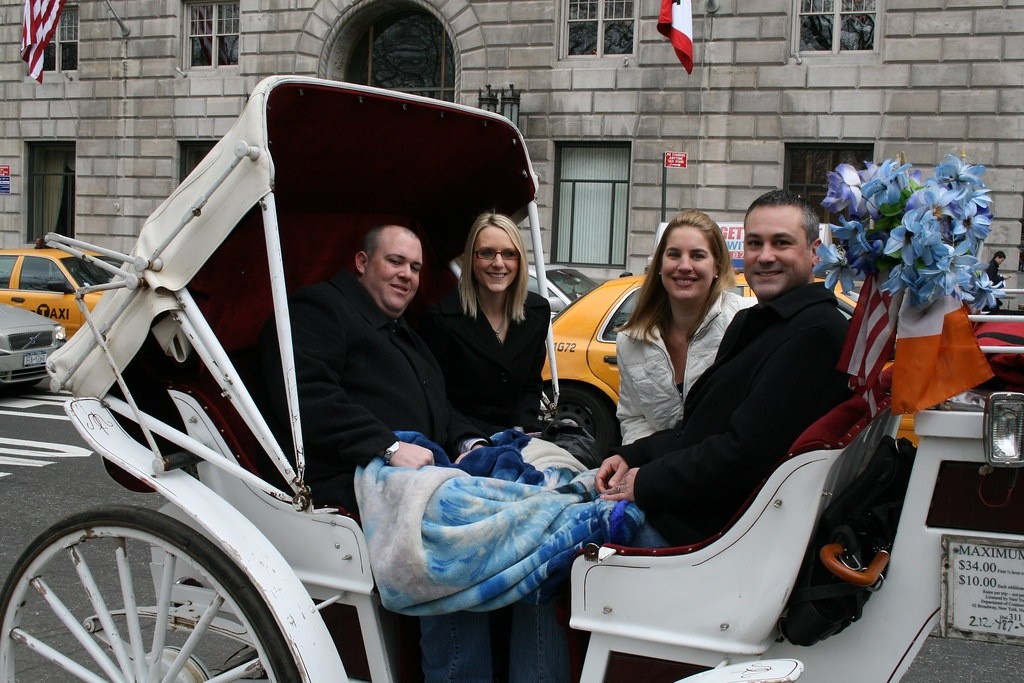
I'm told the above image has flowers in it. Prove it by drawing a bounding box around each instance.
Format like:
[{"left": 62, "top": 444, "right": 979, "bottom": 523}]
[{"left": 808, "top": 153, "right": 1005, "bottom": 312}]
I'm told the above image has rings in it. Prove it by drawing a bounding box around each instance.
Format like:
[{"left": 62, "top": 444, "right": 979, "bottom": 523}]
[{"left": 616, "top": 486, "right": 620, "bottom": 493}]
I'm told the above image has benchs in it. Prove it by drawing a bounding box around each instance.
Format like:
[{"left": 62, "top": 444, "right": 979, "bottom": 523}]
[
  {"left": 563, "top": 394, "right": 890, "bottom": 657},
  {"left": 916, "top": 287, "right": 1024, "bottom": 441},
  {"left": 41, "top": 76, "right": 561, "bottom": 599}
]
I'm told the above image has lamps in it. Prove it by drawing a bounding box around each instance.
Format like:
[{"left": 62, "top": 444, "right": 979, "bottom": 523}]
[
  {"left": 500, "top": 84, "right": 522, "bottom": 131},
  {"left": 478, "top": 85, "right": 498, "bottom": 114}
]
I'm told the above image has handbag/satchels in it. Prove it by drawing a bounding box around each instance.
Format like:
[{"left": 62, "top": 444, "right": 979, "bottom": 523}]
[{"left": 541, "top": 419, "right": 603, "bottom": 469}]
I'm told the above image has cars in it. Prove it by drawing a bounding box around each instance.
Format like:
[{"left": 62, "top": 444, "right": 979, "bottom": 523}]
[
  {"left": 0, "top": 249, "right": 124, "bottom": 339},
  {"left": 528, "top": 264, "right": 598, "bottom": 312},
  {"left": 542, "top": 273, "right": 860, "bottom": 460},
  {"left": 0, "top": 302, "right": 68, "bottom": 383}
]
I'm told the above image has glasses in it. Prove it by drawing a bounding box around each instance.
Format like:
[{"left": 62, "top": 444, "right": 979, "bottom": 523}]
[{"left": 473, "top": 248, "right": 521, "bottom": 260}]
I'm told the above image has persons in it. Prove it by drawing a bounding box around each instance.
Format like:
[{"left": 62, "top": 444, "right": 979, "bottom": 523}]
[
  {"left": 263, "top": 190, "right": 849, "bottom": 683},
  {"left": 982, "top": 251, "right": 1010, "bottom": 313}
]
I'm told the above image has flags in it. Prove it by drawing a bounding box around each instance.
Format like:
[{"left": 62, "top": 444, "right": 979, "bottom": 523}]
[
  {"left": 20, "top": 0, "right": 66, "bottom": 84},
  {"left": 656, "top": 0, "right": 693, "bottom": 74}
]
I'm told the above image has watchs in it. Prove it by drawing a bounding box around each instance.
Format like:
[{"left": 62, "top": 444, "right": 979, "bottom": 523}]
[{"left": 382, "top": 440, "right": 401, "bottom": 465}]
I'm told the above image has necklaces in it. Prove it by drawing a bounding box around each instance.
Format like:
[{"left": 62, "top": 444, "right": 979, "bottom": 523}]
[{"left": 495, "top": 319, "right": 505, "bottom": 336}]
